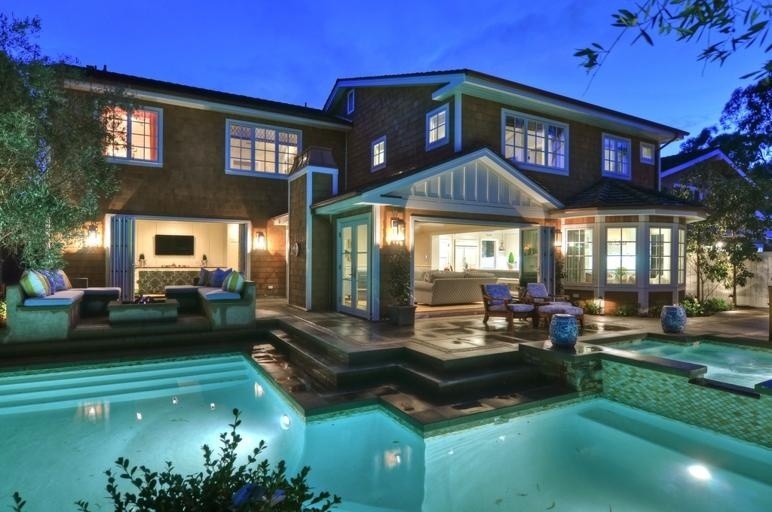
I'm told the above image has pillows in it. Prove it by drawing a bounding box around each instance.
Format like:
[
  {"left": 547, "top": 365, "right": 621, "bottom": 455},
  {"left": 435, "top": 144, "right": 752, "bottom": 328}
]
[
  {"left": 198, "top": 268, "right": 245, "bottom": 292},
  {"left": 20, "top": 269, "right": 72, "bottom": 297}
]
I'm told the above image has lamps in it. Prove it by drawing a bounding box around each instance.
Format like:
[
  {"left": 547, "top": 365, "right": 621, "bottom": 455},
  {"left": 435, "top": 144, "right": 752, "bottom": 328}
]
[
  {"left": 498, "top": 232, "right": 504, "bottom": 251},
  {"left": 390, "top": 217, "right": 406, "bottom": 241},
  {"left": 256, "top": 232, "right": 264, "bottom": 247}
]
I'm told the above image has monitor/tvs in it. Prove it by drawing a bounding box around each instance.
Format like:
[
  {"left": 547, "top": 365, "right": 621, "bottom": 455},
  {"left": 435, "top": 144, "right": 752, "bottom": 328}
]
[{"left": 154, "top": 235, "right": 194, "bottom": 256}]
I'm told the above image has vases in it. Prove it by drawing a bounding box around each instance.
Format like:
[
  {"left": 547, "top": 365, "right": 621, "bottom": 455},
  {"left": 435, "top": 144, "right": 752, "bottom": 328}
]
[
  {"left": 550, "top": 314, "right": 578, "bottom": 347},
  {"left": 661, "top": 305, "right": 686, "bottom": 333}
]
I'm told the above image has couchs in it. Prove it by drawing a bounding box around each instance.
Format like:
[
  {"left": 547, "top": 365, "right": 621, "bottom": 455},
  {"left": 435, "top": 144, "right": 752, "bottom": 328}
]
[
  {"left": 6, "top": 278, "right": 122, "bottom": 340},
  {"left": 415, "top": 270, "right": 498, "bottom": 306},
  {"left": 165, "top": 275, "right": 256, "bottom": 330}
]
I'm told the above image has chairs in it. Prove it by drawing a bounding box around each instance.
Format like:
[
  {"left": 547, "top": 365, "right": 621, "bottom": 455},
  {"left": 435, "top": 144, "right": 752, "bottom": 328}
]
[{"left": 480, "top": 282, "right": 584, "bottom": 331}]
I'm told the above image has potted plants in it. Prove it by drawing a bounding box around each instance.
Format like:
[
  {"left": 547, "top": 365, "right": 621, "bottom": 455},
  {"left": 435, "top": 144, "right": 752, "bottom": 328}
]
[
  {"left": 508, "top": 253, "right": 515, "bottom": 269},
  {"left": 380, "top": 242, "right": 417, "bottom": 327}
]
